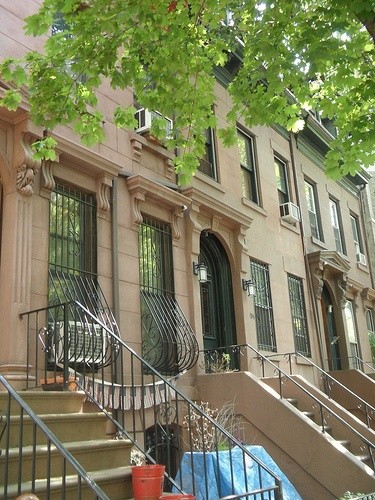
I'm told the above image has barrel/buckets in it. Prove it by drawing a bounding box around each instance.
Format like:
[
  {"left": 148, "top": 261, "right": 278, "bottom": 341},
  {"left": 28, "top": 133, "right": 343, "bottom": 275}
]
[
  {"left": 162, "top": 495, "right": 197, "bottom": 499},
  {"left": 132, "top": 464, "right": 165, "bottom": 500}
]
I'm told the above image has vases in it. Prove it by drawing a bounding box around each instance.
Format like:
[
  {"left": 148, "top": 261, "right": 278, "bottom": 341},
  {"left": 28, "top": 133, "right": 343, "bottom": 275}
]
[{"left": 132, "top": 464, "right": 195, "bottom": 500}]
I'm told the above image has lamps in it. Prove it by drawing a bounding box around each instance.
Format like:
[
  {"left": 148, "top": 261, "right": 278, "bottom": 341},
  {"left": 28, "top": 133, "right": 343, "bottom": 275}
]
[
  {"left": 193, "top": 261, "right": 208, "bottom": 283},
  {"left": 328, "top": 304, "right": 333, "bottom": 313},
  {"left": 242, "top": 278, "right": 255, "bottom": 297}
]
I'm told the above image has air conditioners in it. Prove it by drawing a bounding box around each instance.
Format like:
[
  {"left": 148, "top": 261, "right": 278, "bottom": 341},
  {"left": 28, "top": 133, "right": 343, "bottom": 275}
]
[
  {"left": 134, "top": 107, "right": 173, "bottom": 144},
  {"left": 49, "top": 320, "right": 106, "bottom": 367},
  {"left": 280, "top": 202, "right": 301, "bottom": 224},
  {"left": 355, "top": 253, "right": 367, "bottom": 267}
]
